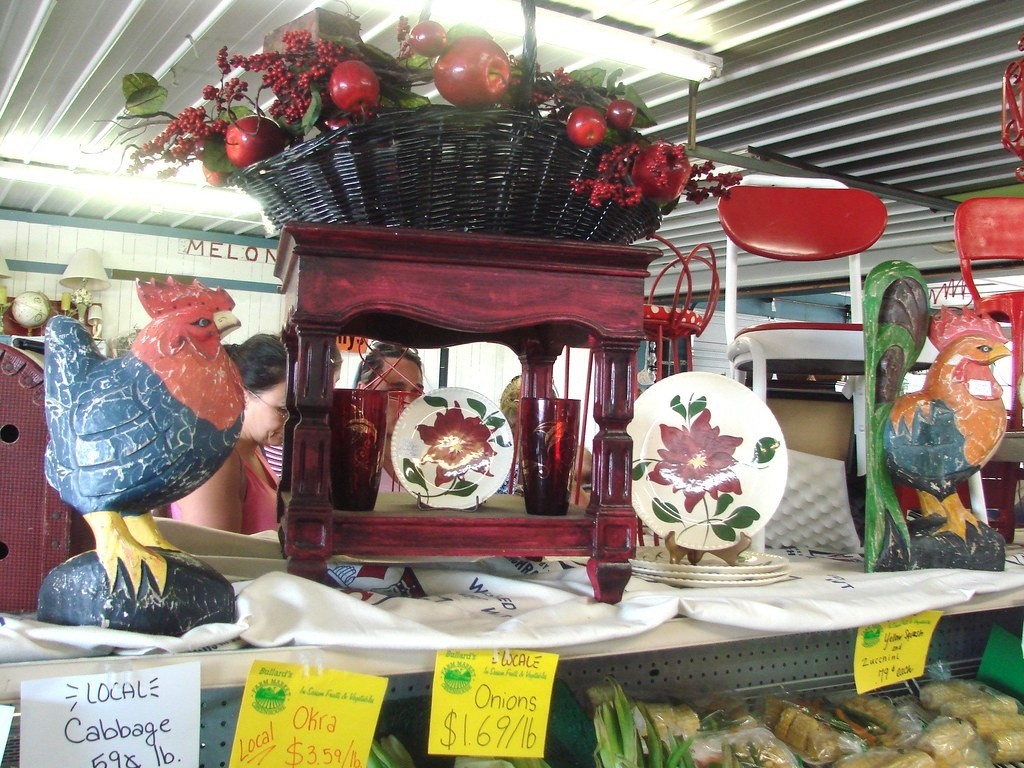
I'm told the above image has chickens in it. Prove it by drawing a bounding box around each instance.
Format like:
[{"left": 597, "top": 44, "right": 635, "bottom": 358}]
[
  {"left": 885, "top": 305, "right": 1016, "bottom": 543},
  {"left": 42, "top": 275, "right": 246, "bottom": 598}
]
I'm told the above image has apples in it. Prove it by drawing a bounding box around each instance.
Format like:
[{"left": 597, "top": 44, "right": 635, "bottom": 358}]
[{"left": 201, "top": 19, "right": 692, "bottom": 200}]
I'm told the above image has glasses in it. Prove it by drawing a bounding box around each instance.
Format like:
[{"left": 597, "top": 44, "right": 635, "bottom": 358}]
[{"left": 247, "top": 389, "right": 289, "bottom": 420}]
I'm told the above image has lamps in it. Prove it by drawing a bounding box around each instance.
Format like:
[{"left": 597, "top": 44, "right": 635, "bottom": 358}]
[
  {"left": 57, "top": 248, "right": 112, "bottom": 342},
  {"left": 0, "top": 251, "right": 12, "bottom": 336}
]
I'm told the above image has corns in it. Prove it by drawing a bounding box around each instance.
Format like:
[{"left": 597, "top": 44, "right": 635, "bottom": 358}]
[{"left": 600, "top": 665, "right": 1024, "bottom": 768}]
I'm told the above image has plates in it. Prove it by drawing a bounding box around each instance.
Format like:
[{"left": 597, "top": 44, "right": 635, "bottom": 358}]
[
  {"left": 626, "top": 546, "right": 791, "bottom": 588},
  {"left": 626, "top": 371, "right": 790, "bottom": 550},
  {"left": 389, "top": 387, "right": 516, "bottom": 510}
]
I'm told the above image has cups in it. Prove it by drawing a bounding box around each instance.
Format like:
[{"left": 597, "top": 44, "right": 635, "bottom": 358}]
[
  {"left": 326, "top": 388, "right": 389, "bottom": 510},
  {"left": 520, "top": 397, "right": 582, "bottom": 515}
]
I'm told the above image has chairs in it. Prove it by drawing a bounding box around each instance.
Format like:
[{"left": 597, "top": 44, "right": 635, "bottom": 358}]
[
  {"left": 718, "top": 174, "right": 886, "bottom": 481},
  {"left": 956, "top": 196, "right": 1024, "bottom": 538}
]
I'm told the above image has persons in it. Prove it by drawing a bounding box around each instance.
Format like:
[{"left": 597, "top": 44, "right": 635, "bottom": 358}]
[
  {"left": 353, "top": 342, "right": 424, "bottom": 495},
  {"left": 495, "top": 376, "right": 593, "bottom": 512},
  {"left": 163, "top": 334, "right": 289, "bottom": 534},
  {"left": 263, "top": 338, "right": 343, "bottom": 482}
]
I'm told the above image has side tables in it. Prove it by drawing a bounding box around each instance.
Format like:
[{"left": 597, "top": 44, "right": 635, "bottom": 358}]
[{"left": 270, "top": 219, "right": 665, "bottom": 606}]
